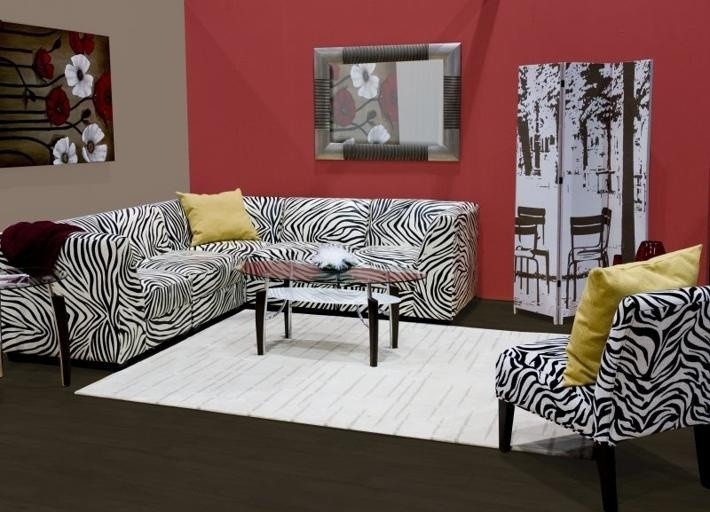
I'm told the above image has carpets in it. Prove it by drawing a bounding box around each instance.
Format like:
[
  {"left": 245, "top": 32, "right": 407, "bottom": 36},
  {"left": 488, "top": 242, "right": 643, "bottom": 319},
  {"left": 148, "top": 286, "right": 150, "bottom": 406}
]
[{"left": 73, "top": 307, "right": 596, "bottom": 461}]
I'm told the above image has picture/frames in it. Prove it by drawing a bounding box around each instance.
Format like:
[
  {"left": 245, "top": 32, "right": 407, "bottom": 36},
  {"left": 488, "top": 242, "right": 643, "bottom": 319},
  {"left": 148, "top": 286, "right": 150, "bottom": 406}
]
[{"left": 312, "top": 42, "right": 462, "bottom": 163}]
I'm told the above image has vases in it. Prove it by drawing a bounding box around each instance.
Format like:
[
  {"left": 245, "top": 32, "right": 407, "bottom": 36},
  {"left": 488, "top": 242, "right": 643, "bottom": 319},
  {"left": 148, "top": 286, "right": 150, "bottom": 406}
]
[{"left": 318, "top": 261, "right": 352, "bottom": 274}]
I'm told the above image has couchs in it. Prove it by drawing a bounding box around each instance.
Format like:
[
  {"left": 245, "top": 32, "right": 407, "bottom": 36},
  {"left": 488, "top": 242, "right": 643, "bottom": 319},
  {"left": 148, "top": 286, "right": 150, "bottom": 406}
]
[{"left": 1, "top": 193, "right": 480, "bottom": 365}]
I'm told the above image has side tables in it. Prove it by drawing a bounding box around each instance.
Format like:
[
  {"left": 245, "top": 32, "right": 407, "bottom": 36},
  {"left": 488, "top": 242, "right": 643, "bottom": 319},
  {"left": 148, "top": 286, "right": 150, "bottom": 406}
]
[{"left": 1, "top": 269, "right": 72, "bottom": 388}]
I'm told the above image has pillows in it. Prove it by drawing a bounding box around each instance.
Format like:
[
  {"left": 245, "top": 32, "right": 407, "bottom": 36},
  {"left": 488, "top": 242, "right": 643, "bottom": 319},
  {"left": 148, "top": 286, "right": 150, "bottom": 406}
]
[
  {"left": 175, "top": 186, "right": 262, "bottom": 247},
  {"left": 561, "top": 242, "right": 704, "bottom": 389}
]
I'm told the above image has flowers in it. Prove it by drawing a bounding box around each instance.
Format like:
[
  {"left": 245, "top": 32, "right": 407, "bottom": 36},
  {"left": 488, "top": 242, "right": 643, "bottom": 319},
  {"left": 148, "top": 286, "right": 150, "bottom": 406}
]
[
  {"left": 0, "top": 20, "right": 113, "bottom": 166},
  {"left": 329, "top": 63, "right": 398, "bottom": 145}
]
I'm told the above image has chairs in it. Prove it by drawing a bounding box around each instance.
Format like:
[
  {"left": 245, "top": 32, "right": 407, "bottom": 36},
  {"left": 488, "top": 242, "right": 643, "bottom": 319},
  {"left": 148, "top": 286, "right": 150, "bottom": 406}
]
[
  {"left": 565, "top": 207, "right": 612, "bottom": 308},
  {"left": 515, "top": 206, "right": 550, "bottom": 305},
  {"left": 494, "top": 286, "right": 710, "bottom": 511}
]
